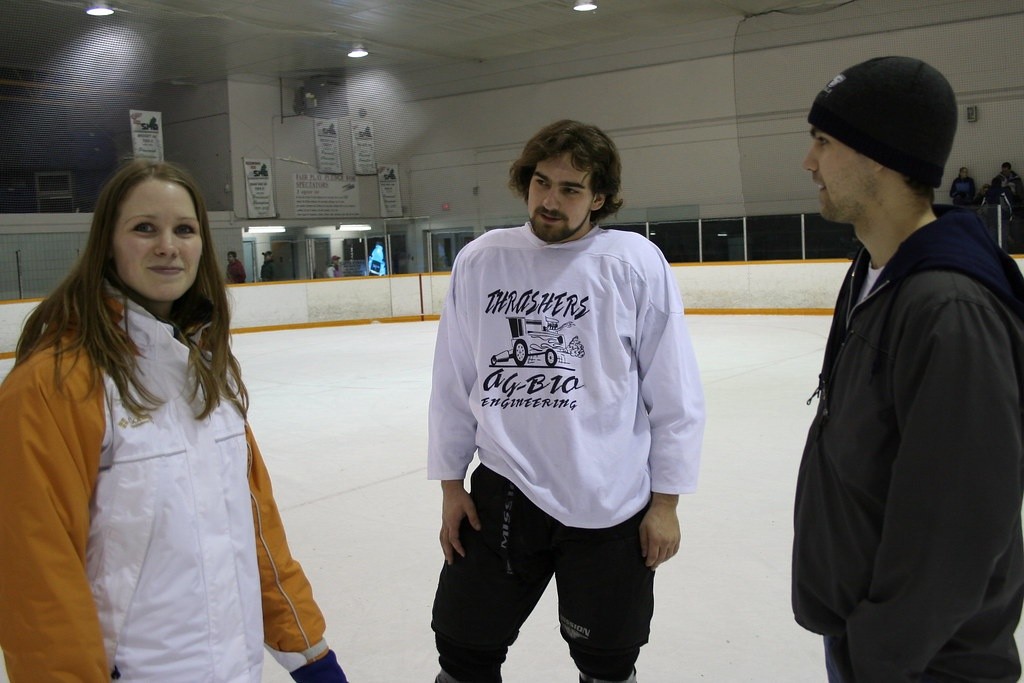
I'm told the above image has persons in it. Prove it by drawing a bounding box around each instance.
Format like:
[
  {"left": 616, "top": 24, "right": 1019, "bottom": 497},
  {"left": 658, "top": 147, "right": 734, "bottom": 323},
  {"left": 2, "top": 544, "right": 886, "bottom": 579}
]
[
  {"left": 430, "top": 116, "right": 703, "bottom": 683},
  {"left": 225, "top": 251, "right": 246, "bottom": 284},
  {"left": 0, "top": 159, "right": 352, "bottom": 683},
  {"left": 949, "top": 162, "right": 1024, "bottom": 255},
  {"left": 792, "top": 55, "right": 1024, "bottom": 682},
  {"left": 326, "top": 255, "right": 342, "bottom": 277},
  {"left": 261, "top": 251, "right": 275, "bottom": 282}
]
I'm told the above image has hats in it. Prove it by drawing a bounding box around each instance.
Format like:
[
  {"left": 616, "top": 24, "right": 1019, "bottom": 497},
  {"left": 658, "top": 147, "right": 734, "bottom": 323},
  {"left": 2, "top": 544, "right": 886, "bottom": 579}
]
[
  {"left": 808, "top": 56, "right": 959, "bottom": 189},
  {"left": 332, "top": 256, "right": 340, "bottom": 260},
  {"left": 262, "top": 251, "right": 272, "bottom": 256}
]
